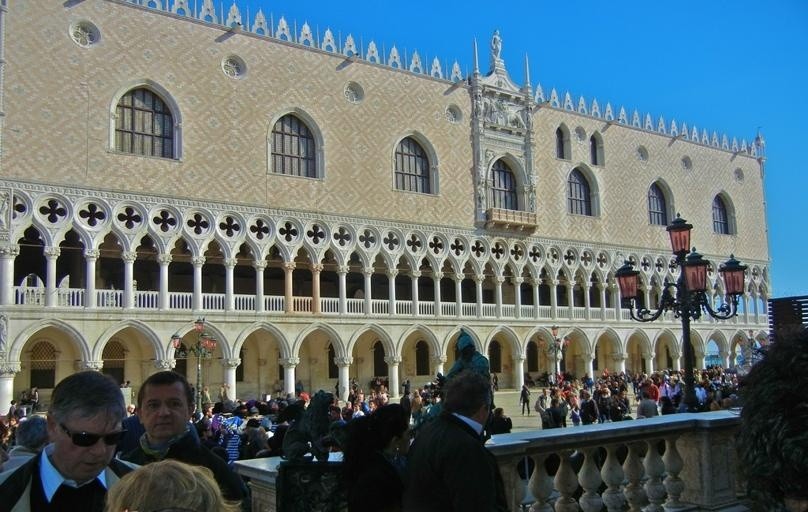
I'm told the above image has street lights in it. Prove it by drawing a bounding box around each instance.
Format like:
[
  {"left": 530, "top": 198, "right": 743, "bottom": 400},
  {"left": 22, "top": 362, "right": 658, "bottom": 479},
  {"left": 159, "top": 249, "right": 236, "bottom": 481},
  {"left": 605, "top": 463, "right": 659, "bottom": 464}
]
[
  {"left": 171, "top": 317, "right": 219, "bottom": 416},
  {"left": 615, "top": 208, "right": 751, "bottom": 415},
  {"left": 536, "top": 323, "right": 571, "bottom": 390}
]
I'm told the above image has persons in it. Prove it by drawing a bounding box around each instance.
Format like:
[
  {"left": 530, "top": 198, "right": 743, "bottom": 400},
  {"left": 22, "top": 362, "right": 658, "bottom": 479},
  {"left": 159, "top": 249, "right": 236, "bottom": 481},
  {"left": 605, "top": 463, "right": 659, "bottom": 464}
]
[
  {"left": 1, "top": 369, "right": 514, "bottom": 511},
  {"left": 437, "top": 333, "right": 495, "bottom": 415},
  {"left": 520, "top": 364, "right": 739, "bottom": 429}
]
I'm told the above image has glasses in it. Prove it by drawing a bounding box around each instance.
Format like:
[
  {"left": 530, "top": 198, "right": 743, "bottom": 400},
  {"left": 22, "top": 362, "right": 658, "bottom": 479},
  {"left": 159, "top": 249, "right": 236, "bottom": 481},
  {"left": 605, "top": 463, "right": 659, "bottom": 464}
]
[{"left": 59, "top": 423, "right": 123, "bottom": 446}]
